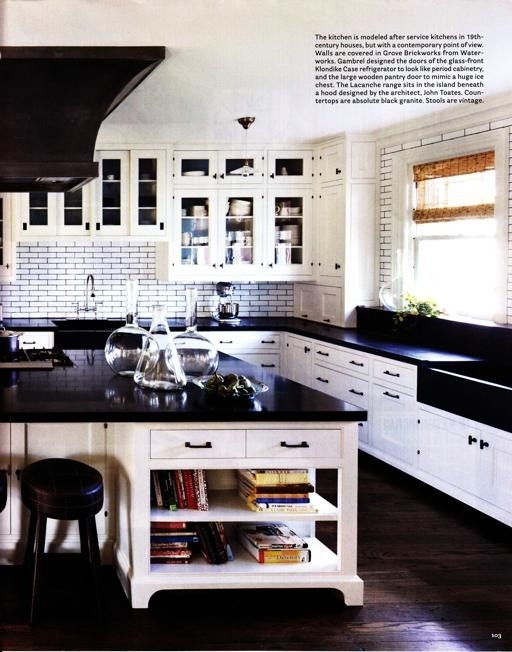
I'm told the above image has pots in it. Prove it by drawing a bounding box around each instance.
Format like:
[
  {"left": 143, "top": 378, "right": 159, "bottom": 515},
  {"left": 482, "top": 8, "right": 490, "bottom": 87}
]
[
  {"left": 219, "top": 303, "right": 239, "bottom": 319},
  {"left": 0, "top": 332, "right": 22, "bottom": 358}
]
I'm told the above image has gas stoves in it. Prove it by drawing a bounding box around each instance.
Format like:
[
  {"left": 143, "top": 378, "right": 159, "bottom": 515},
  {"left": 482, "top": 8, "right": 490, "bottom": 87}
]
[{"left": 0, "top": 346, "right": 74, "bottom": 368}]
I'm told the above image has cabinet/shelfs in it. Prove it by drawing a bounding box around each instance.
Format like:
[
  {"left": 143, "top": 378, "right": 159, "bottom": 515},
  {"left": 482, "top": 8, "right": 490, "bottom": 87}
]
[
  {"left": 0, "top": 422, "right": 110, "bottom": 569},
  {"left": 17, "top": 331, "right": 55, "bottom": 351},
  {"left": 90, "top": 149, "right": 168, "bottom": 243},
  {"left": 156, "top": 150, "right": 316, "bottom": 284},
  {"left": 112, "top": 421, "right": 366, "bottom": 612},
  {"left": 318, "top": 138, "right": 380, "bottom": 286},
  {"left": 142, "top": 331, "right": 214, "bottom": 352},
  {"left": 0, "top": 191, "right": 16, "bottom": 284},
  {"left": 415, "top": 409, "right": 512, "bottom": 527},
  {"left": 284, "top": 335, "right": 315, "bottom": 391},
  {"left": 16, "top": 187, "right": 90, "bottom": 244},
  {"left": 292, "top": 282, "right": 381, "bottom": 330},
  {"left": 370, "top": 357, "right": 415, "bottom": 472},
  {"left": 211, "top": 332, "right": 282, "bottom": 378},
  {"left": 313, "top": 342, "right": 369, "bottom": 455}
]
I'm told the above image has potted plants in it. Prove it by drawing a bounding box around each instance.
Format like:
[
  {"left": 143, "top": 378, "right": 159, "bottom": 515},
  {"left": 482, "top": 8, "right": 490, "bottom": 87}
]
[{"left": 390, "top": 293, "right": 446, "bottom": 326}]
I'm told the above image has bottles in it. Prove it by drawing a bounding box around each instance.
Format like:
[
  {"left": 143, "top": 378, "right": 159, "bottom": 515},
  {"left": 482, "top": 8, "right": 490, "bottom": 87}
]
[{"left": 102, "top": 279, "right": 218, "bottom": 392}]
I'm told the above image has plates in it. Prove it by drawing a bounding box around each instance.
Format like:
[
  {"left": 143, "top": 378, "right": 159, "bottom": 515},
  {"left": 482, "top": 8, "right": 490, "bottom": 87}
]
[
  {"left": 188, "top": 374, "right": 271, "bottom": 398},
  {"left": 178, "top": 169, "right": 301, "bottom": 269}
]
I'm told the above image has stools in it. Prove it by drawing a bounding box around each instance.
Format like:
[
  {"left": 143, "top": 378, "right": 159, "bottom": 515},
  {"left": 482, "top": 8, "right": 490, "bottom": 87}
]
[{"left": 17, "top": 456, "right": 109, "bottom": 629}]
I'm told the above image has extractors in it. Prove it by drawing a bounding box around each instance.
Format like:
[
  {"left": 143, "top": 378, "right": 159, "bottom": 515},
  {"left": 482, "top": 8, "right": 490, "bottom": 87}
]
[
  {"left": 213, "top": 316, "right": 241, "bottom": 324},
  {"left": 0, "top": 45, "right": 167, "bottom": 193}
]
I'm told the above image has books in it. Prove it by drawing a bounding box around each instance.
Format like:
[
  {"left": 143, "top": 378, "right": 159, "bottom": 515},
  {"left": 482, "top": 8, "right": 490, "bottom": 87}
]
[{"left": 150, "top": 470, "right": 319, "bottom": 564}]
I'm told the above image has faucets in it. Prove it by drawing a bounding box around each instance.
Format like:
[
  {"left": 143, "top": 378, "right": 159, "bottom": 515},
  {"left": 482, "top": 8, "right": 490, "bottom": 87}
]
[{"left": 85, "top": 273, "right": 95, "bottom": 310}]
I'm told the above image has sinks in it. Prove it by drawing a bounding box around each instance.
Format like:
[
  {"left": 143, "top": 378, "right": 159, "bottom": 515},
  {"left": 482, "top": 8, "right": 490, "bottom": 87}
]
[{"left": 52, "top": 319, "right": 125, "bottom": 350}]
